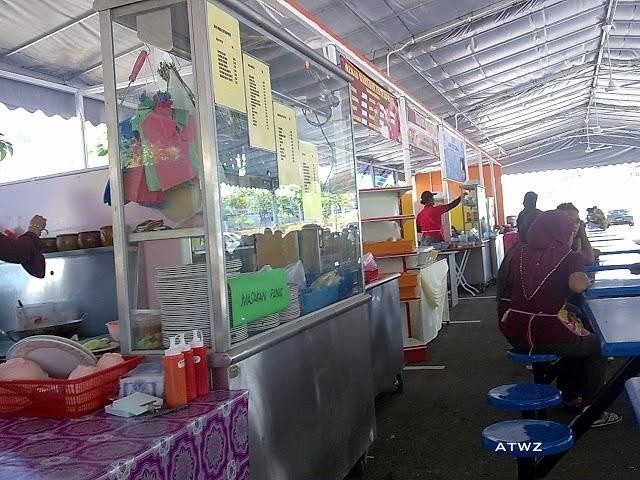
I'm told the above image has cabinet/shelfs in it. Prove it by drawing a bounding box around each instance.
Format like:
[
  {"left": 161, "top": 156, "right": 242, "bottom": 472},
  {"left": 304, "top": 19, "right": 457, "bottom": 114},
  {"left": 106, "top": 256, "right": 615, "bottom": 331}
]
[
  {"left": 1, "top": 3, "right": 375, "bottom": 479},
  {"left": 357, "top": 185, "right": 427, "bottom": 364},
  {"left": 459, "top": 184, "right": 488, "bottom": 244}
]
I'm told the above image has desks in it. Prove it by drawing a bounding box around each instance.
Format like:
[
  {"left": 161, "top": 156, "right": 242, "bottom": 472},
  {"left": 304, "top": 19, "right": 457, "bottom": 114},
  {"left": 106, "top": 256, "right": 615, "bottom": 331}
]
[
  {"left": 440, "top": 243, "right": 485, "bottom": 296},
  {"left": 482, "top": 224, "right": 640, "bottom": 480}
]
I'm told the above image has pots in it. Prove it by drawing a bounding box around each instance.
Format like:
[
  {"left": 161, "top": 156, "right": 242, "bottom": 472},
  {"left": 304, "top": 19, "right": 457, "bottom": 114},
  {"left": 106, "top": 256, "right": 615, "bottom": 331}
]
[{"left": 0, "top": 314, "right": 88, "bottom": 342}]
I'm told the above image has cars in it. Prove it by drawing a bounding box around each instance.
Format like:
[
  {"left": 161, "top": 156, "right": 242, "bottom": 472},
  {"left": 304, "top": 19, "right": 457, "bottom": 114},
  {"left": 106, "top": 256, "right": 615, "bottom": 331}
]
[
  {"left": 195, "top": 229, "right": 247, "bottom": 254},
  {"left": 224, "top": 205, "right": 351, "bottom": 222},
  {"left": 607, "top": 207, "right": 638, "bottom": 229}
]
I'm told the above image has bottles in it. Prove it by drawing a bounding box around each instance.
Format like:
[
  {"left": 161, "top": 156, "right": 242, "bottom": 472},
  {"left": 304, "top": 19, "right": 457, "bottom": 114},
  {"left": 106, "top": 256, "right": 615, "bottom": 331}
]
[{"left": 162, "top": 330, "right": 209, "bottom": 410}]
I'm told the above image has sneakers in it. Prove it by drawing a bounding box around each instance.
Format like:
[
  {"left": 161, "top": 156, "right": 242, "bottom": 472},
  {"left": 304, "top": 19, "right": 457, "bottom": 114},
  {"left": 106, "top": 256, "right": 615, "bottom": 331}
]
[{"left": 590, "top": 410, "right": 621, "bottom": 428}]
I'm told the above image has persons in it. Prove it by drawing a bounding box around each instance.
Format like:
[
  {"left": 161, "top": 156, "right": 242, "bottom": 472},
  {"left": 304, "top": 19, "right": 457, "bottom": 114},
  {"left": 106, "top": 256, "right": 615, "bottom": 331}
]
[
  {"left": 0, "top": 215, "right": 47, "bottom": 278},
  {"left": 416, "top": 191, "right": 468, "bottom": 246},
  {"left": 495, "top": 192, "right": 622, "bottom": 428}
]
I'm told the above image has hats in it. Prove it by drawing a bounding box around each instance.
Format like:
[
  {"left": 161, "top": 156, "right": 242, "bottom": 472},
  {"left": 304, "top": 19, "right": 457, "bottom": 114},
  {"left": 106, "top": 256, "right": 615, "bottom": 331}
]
[{"left": 421, "top": 191, "right": 437, "bottom": 204}]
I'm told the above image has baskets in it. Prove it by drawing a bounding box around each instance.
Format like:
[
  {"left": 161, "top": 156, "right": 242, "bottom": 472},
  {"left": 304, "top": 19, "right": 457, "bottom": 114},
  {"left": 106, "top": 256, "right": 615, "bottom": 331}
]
[{"left": 0, "top": 354, "right": 145, "bottom": 419}]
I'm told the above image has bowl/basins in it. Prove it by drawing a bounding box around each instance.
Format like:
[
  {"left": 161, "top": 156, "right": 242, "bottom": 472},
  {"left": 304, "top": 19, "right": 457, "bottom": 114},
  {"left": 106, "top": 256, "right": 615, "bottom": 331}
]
[
  {"left": 105, "top": 319, "right": 161, "bottom": 343},
  {"left": 39, "top": 226, "right": 113, "bottom": 252},
  {"left": 0, "top": 353, "right": 127, "bottom": 412}
]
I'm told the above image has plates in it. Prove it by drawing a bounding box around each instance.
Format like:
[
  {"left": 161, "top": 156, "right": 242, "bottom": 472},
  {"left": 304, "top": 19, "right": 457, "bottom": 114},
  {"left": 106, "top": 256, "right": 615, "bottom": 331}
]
[
  {"left": 151, "top": 246, "right": 301, "bottom": 347},
  {"left": 6, "top": 335, "right": 120, "bottom": 378}
]
